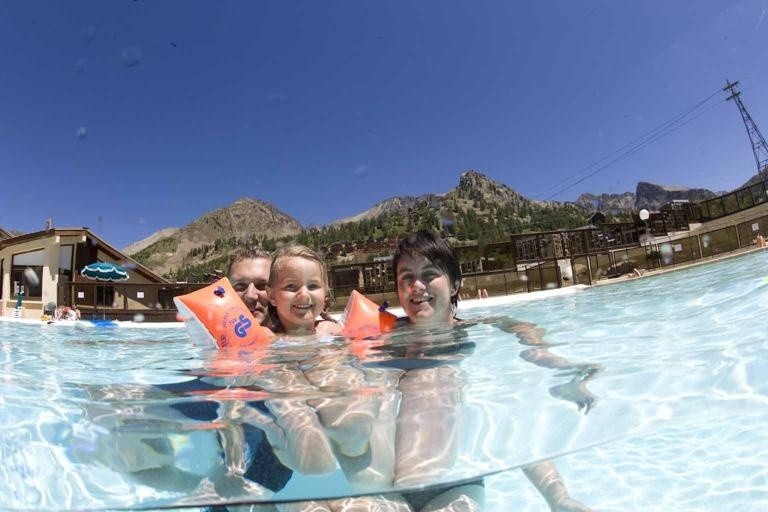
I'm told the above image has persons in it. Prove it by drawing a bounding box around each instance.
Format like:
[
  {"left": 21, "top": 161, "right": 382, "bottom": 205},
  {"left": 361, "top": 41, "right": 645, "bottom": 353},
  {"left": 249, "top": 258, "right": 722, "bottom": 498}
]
[
  {"left": 392, "top": 230, "right": 462, "bottom": 320},
  {"left": 226, "top": 247, "right": 284, "bottom": 334},
  {"left": 266, "top": 245, "right": 342, "bottom": 335},
  {"left": 55, "top": 304, "right": 81, "bottom": 321}
]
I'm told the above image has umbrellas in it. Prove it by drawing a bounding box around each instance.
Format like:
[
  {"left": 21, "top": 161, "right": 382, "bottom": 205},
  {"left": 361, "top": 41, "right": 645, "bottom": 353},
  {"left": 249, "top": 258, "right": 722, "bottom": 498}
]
[{"left": 81, "top": 262, "right": 130, "bottom": 320}]
